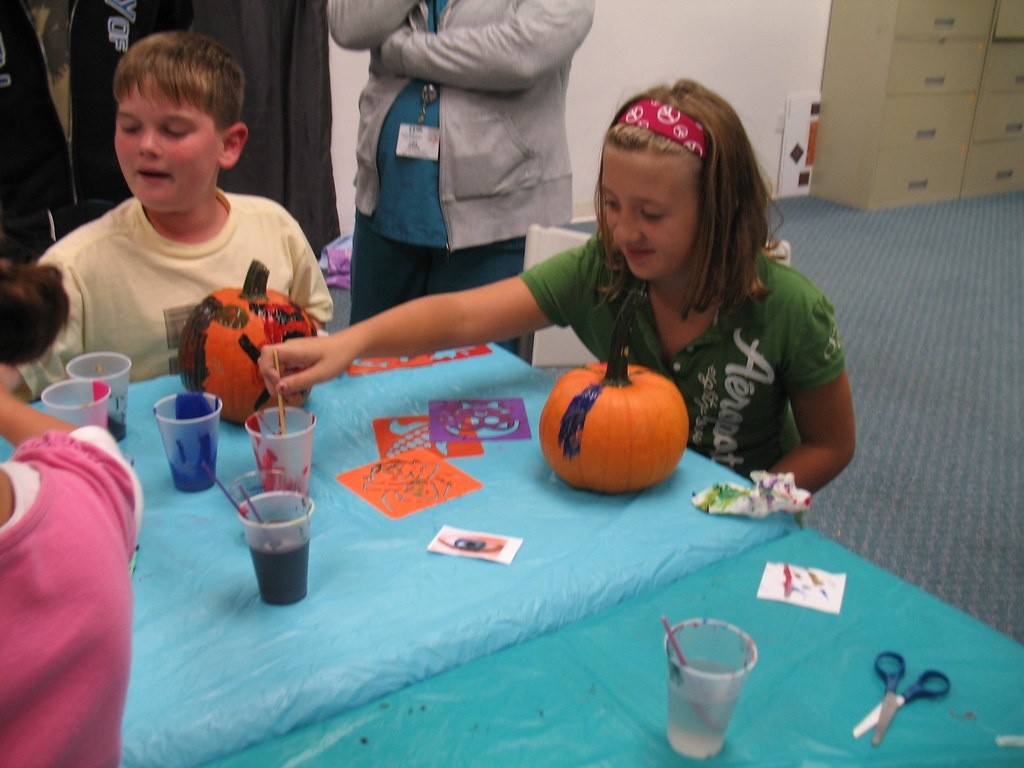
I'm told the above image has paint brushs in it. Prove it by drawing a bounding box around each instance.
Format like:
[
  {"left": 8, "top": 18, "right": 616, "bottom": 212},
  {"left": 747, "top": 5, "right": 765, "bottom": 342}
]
[
  {"left": 661, "top": 616, "right": 715, "bottom": 730},
  {"left": 201, "top": 461, "right": 250, "bottom": 523},
  {"left": 238, "top": 486, "right": 264, "bottom": 523},
  {"left": 274, "top": 350, "right": 285, "bottom": 435}
]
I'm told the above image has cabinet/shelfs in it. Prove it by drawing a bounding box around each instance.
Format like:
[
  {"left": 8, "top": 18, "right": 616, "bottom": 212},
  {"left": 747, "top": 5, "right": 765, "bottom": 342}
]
[{"left": 809, "top": 0, "right": 1024, "bottom": 210}]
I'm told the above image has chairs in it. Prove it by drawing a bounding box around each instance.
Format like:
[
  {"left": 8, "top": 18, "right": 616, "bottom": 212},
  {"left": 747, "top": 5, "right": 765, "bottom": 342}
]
[{"left": 516, "top": 219, "right": 599, "bottom": 371}]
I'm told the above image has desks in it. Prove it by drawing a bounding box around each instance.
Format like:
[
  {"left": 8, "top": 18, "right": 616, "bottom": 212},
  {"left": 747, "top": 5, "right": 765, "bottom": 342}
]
[
  {"left": 0, "top": 338, "right": 799, "bottom": 768},
  {"left": 196, "top": 526, "right": 1024, "bottom": 768}
]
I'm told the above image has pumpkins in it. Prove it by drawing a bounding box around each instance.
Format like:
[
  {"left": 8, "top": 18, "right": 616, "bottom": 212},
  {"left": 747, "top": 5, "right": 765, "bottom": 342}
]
[
  {"left": 177, "top": 261, "right": 317, "bottom": 423},
  {"left": 538, "top": 290, "right": 689, "bottom": 493}
]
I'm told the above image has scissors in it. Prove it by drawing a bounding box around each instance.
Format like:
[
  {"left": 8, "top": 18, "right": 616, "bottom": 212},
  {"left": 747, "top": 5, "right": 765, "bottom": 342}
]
[{"left": 851, "top": 651, "right": 950, "bottom": 747}]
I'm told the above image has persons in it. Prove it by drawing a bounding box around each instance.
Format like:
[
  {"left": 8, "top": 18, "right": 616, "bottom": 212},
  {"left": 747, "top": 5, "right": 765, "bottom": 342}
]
[
  {"left": 327, "top": 0, "right": 595, "bottom": 364},
  {"left": 0, "top": 32, "right": 334, "bottom": 400},
  {"left": 0, "top": 240, "right": 142, "bottom": 768},
  {"left": 260, "top": 80, "right": 856, "bottom": 496}
]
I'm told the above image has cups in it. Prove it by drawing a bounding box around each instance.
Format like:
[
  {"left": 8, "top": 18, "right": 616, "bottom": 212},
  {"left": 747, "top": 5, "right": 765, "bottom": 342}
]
[
  {"left": 664, "top": 618, "right": 758, "bottom": 757},
  {"left": 154, "top": 392, "right": 223, "bottom": 492},
  {"left": 237, "top": 490, "right": 315, "bottom": 605},
  {"left": 244, "top": 406, "right": 316, "bottom": 498},
  {"left": 65, "top": 351, "right": 132, "bottom": 443},
  {"left": 41, "top": 380, "right": 112, "bottom": 434}
]
